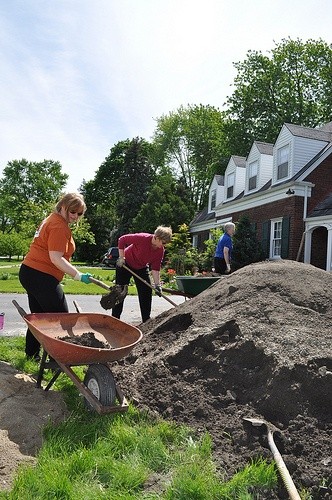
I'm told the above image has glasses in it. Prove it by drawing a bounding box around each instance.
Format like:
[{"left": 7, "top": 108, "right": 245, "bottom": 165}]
[{"left": 69, "top": 210, "right": 83, "bottom": 216}]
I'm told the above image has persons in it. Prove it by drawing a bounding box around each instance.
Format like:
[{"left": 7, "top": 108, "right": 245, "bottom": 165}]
[
  {"left": 213, "top": 222, "right": 236, "bottom": 276},
  {"left": 18, "top": 193, "right": 96, "bottom": 362},
  {"left": 111, "top": 225, "right": 173, "bottom": 323}
]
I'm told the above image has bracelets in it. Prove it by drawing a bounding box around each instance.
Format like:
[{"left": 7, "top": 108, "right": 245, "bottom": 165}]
[{"left": 119, "top": 256, "right": 125, "bottom": 259}]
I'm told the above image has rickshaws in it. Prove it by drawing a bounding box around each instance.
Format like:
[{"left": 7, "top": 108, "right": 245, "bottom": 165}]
[
  {"left": 12, "top": 299, "right": 143, "bottom": 415},
  {"left": 162, "top": 276, "right": 221, "bottom": 301}
]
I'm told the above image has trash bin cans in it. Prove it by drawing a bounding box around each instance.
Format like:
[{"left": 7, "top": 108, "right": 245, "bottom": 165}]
[{"left": 0, "top": 312, "right": 4, "bottom": 329}]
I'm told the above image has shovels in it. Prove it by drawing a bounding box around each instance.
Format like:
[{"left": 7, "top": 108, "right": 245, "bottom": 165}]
[
  {"left": 88, "top": 276, "right": 129, "bottom": 305},
  {"left": 242, "top": 416, "right": 301, "bottom": 500}
]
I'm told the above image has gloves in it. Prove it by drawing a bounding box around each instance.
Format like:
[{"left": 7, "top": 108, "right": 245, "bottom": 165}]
[
  {"left": 225, "top": 264, "right": 231, "bottom": 273},
  {"left": 116, "top": 256, "right": 126, "bottom": 268},
  {"left": 73, "top": 272, "right": 95, "bottom": 284},
  {"left": 155, "top": 285, "right": 162, "bottom": 297}
]
